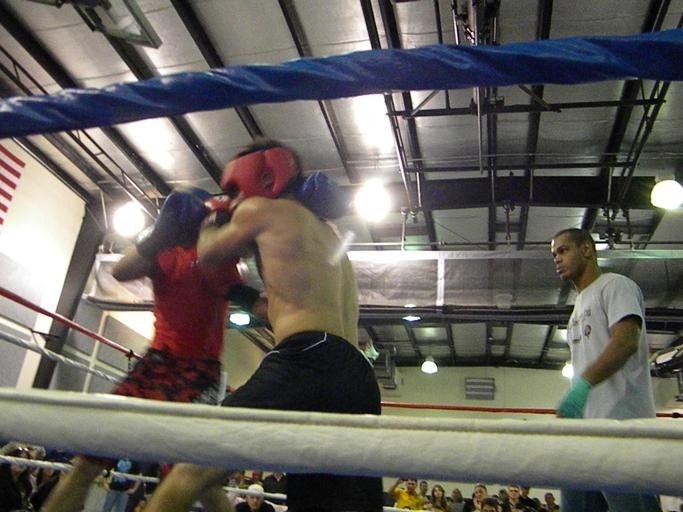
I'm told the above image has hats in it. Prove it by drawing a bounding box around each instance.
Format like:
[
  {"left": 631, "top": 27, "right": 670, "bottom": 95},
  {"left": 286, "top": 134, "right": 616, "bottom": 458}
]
[{"left": 245, "top": 484, "right": 265, "bottom": 496}]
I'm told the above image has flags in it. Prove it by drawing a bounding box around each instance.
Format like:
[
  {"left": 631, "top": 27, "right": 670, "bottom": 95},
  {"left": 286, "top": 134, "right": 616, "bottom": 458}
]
[{"left": 0, "top": 143, "right": 26, "bottom": 224}]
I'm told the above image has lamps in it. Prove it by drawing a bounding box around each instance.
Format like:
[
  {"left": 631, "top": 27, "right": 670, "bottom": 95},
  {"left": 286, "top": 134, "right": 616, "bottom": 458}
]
[
  {"left": 650, "top": 158, "right": 682, "bottom": 211},
  {"left": 562, "top": 359, "right": 574, "bottom": 378},
  {"left": 354, "top": 170, "right": 391, "bottom": 222},
  {"left": 110, "top": 199, "right": 148, "bottom": 237},
  {"left": 421, "top": 345, "right": 438, "bottom": 374}
]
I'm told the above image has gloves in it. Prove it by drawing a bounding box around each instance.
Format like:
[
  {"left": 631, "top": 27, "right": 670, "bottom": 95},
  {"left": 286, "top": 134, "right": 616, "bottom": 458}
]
[
  {"left": 555, "top": 379, "right": 593, "bottom": 421},
  {"left": 300, "top": 171, "right": 352, "bottom": 220},
  {"left": 136, "top": 184, "right": 230, "bottom": 258}
]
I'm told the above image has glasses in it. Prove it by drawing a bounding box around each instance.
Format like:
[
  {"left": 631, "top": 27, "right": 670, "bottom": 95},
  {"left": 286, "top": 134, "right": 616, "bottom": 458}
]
[{"left": 11, "top": 446, "right": 31, "bottom": 458}]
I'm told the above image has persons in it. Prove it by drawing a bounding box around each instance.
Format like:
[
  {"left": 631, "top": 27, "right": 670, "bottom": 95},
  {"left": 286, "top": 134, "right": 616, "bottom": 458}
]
[
  {"left": 39, "top": 170, "right": 348, "bottom": 511},
  {"left": 141, "top": 137, "right": 385, "bottom": 511},
  {"left": 549, "top": 228, "right": 664, "bottom": 511}
]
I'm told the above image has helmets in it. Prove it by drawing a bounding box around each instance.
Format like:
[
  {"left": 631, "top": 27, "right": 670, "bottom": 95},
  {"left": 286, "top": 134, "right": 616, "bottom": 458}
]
[{"left": 220, "top": 144, "right": 301, "bottom": 216}]
